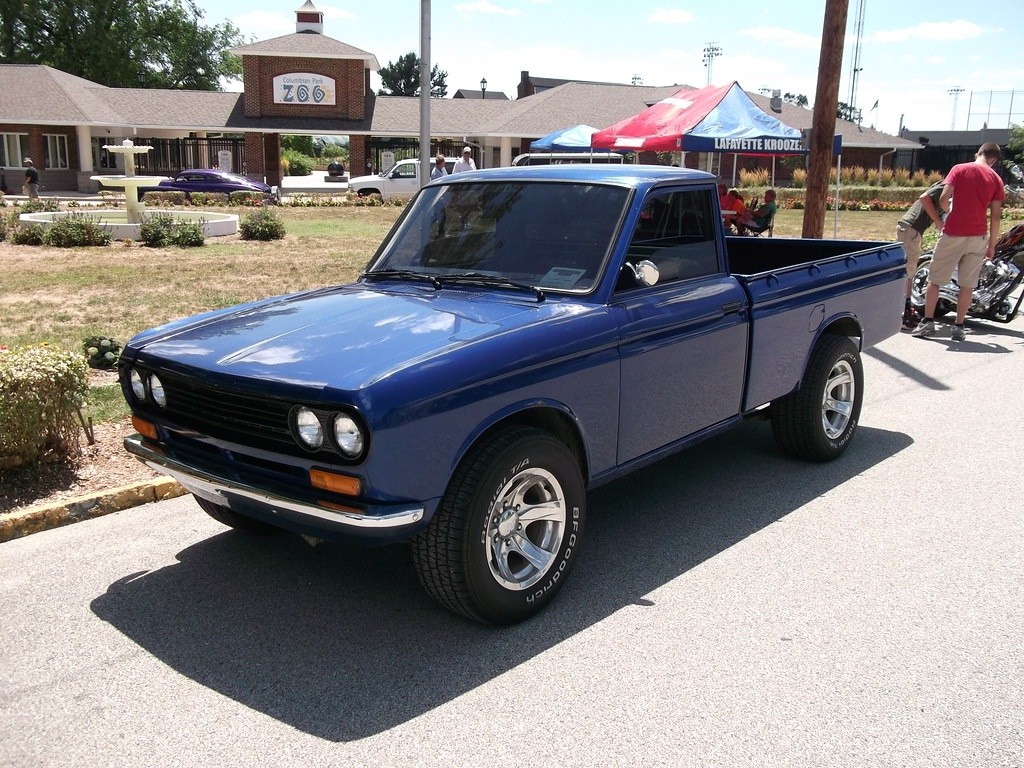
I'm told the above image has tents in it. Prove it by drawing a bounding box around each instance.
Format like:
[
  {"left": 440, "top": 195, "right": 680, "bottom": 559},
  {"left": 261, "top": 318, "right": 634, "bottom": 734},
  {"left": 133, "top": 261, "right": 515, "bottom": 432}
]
[
  {"left": 590, "top": 79, "right": 843, "bottom": 237},
  {"left": 528, "top": 122, "right": 635, "bottom": 164}
]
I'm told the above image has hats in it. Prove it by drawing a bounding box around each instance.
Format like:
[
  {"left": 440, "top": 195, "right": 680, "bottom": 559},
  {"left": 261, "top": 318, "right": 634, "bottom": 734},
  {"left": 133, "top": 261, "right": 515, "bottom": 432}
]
[{"left": 462, "top": 146, "right": 471, "bottom": 154}]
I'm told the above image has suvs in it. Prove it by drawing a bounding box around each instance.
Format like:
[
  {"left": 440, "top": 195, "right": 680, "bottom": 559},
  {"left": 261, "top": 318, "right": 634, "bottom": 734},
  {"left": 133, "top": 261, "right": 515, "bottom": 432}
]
[{"left": 344, "top": 157, "right": 461, "bottom": 203}]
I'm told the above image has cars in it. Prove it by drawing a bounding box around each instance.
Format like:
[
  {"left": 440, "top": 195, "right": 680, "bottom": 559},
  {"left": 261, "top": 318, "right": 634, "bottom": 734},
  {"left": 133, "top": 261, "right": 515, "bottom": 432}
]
[{"left": 157, "top": 168, "right": 271, "bottom": 199}]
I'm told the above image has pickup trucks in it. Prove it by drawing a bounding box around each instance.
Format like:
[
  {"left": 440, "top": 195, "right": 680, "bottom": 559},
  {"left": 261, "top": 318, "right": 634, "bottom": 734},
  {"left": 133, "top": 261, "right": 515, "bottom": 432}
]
[{"left": 117, "top": 163, "right": 908, "bottom": 628}]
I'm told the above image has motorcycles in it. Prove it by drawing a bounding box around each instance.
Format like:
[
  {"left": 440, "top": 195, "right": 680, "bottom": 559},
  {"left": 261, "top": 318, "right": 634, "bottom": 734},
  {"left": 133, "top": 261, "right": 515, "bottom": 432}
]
[{"left": 910, "top": 164, "right": 1024, "bottom": 324}]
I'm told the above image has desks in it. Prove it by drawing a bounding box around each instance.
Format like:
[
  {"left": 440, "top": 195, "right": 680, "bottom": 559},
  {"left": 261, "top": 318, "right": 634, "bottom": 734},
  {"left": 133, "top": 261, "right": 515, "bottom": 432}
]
[{"left": 721, "top": 210, "right": 737, "bottom": 215}]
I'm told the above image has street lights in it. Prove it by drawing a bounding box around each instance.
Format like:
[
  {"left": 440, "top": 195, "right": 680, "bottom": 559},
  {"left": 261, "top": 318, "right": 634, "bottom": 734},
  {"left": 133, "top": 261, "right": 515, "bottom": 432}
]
[{"left": 480, "top": 77, "right": 487, "bottom": 98}]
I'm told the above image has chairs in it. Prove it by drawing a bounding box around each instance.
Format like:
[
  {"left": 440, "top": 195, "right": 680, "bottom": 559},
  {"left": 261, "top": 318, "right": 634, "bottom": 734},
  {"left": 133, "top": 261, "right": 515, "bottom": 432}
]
[
  {"left": 741, "top": 209, "right": 775, "bottom": 238},
  {"left": 749, "top": 196, "right": 758, "bottom": 209}
]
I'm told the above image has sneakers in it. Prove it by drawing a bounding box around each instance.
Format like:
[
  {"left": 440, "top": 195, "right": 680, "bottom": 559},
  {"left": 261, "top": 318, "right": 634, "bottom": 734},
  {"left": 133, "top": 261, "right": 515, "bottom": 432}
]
[
  {"left": 899, "top": 315, "right": 917, "bottom": 332},
  {"left": 911, "top": 321, "right": 935, "bottom": 338},
  {"left": 950, "top": 324, "right": 965, "bottom": 341}
]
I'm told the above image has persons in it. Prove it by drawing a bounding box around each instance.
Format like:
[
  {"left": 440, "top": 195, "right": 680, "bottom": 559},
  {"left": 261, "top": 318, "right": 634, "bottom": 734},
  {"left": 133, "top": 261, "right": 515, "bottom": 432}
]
[
  {"left": 895, "top": 179, "right": 956, "bottom": 329},
  {"left": 429, "top": 155, "right": 449, "bottom": 235},
  {"left": 734, "top": 189, "right": 778, "bottom": 236},
  {"left": 717, "top": 183, "right": 730, "bottom": 210},
  {"left": 452, "top": 146, "right": 477, "bottom": 230},
  {"left": 23, "top": 157, "right": 40, "bottom": 198},
  {"left": 911, "top": 140, "right": 1007, "bottom": 339},
  {"left": 724, "top": 191, "right": 746, "bottom": 235}
]
